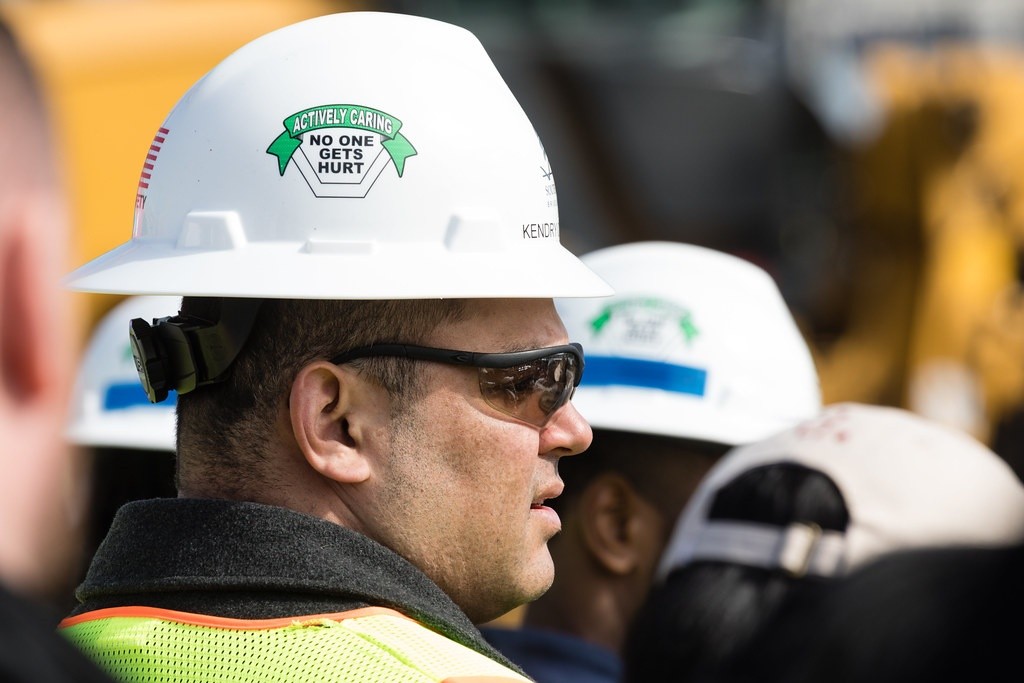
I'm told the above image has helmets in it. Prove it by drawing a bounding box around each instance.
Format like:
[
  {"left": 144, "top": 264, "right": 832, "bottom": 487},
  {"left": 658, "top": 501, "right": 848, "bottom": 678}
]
[
  {"left": 553, "top": 241, "right": 823, "bottom": 446},
  {"left": 66, "top": 12, "right": 615, "bottom": 301},
  {"left": 68, "top": 296, "right": 182, "bottom": 452}
]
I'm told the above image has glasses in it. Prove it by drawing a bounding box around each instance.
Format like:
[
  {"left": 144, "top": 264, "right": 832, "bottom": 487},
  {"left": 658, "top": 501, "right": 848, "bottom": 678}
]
[{"left": 287, "top": 343, "right": 584, "bottom": 426}]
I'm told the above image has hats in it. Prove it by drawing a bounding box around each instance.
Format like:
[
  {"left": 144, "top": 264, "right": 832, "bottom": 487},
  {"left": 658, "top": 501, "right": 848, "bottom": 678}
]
[{"left": 657, "top": 403, "right": 1024, "bottom": 577}]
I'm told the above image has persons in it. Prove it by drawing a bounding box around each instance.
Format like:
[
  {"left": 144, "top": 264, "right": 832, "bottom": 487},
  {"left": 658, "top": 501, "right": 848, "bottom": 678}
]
[
  {"left": 1, "top": 18, "right": 110, "bottom": 683},
  {"left": 59, "top": 10, "right": 613, "bottom": 682},
  {"left": 64, "top": 294, "right": 182, "bottom": 559},
  {"left": 621, "top": 399, "right": 1024, "bottom": 682},
  {"left": 475, "top": 241, "right": 823, "bottom": 682}
]
